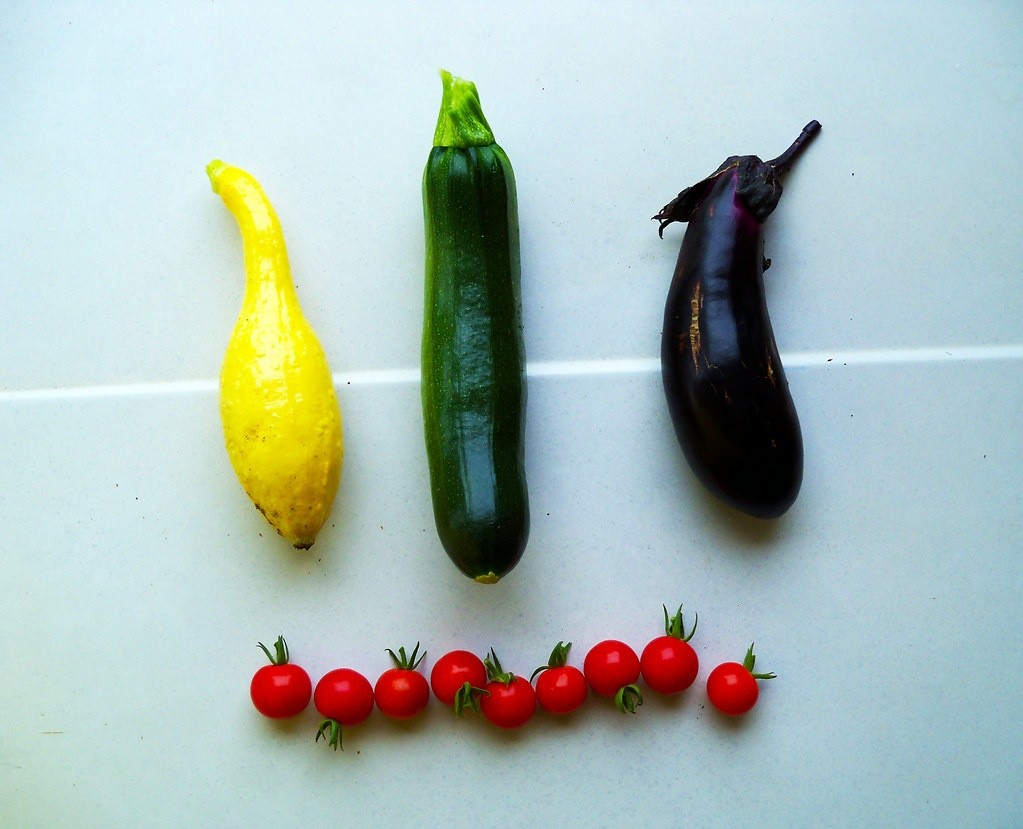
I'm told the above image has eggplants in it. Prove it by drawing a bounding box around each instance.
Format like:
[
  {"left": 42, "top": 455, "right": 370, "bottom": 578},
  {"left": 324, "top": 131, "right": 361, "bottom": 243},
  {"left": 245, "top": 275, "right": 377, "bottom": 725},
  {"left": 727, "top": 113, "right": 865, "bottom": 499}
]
[{"left": 653, "top": 120, "right": 820, "bottom": 519}]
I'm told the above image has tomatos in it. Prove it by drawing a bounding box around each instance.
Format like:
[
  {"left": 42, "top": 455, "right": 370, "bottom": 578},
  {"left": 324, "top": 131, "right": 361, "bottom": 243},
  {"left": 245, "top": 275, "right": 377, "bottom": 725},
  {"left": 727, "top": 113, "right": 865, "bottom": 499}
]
[{"left": 251, "top": 604, "right": 776, "bottom": 751}]
